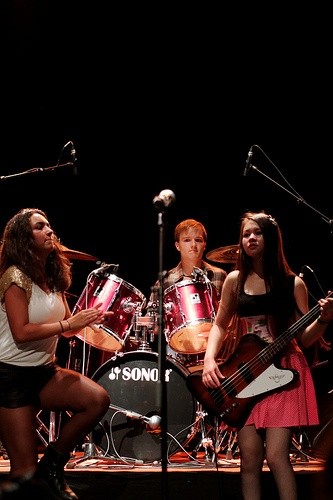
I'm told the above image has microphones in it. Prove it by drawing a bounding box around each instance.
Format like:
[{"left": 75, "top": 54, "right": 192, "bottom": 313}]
[
  {"left": 193, "top": 266, "right": 211, "bottom": 284},
  {"left": 71, "top": 144, "right": 78, "bottom": 175},
  {"left": 153, "top": 188, "right": 176, "bottom": 210},
  {"left": 92, "top": 264, "right": 119, "bottom": 276},
  {"left": 243, "top": 150, "right": 253, "bottom": 177}
]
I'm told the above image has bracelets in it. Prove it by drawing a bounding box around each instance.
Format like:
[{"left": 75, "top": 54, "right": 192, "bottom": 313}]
[
  {"left": 59, "top": 321, "right": 64, "bottom": 332},
  {"left": 316, "top": 317, "right": 325, "bottom": 324},
  {"left": 67, "top": 320, "right": 70, "bottom": 329}
]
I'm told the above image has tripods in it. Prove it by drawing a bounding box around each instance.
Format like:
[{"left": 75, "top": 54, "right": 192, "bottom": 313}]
[{"left": 65, "top": 271, "right": 309, "bottom": 470}]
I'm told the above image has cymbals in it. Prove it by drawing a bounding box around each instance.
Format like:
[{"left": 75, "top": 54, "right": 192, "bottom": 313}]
[
  {"left": 60, "top": 244, "right": 98, "bottom": 260},
  {"left": 206, "top": 245, "right": 240, "bottom": 264}
]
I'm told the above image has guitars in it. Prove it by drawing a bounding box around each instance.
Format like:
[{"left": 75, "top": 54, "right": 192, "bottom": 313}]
[{"left": 185, "top": 291, "right": 333, "bottom": 429}]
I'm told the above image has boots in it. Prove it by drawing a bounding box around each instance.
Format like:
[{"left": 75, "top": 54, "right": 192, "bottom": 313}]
[{"left": 37, "top": 441, "right": 78, "bottom": 500}]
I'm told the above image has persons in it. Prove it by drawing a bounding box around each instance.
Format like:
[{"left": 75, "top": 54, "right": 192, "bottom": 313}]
[
  {"left": 0, "top": 209, "right": 114, "bottom": 500},
  {"left": 149, "top": 220, "right": 229, "bottom": 356},
  {"left": 202, "top": 213, "right": 333, "bottom": 500}
]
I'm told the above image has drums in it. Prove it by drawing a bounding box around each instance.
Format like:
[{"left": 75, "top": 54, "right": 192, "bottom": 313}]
[
  {"left": 163, "top": 279, "right": 229, "bottom": 354},
  {"left": 88, "top": 351, "right": 202, "bottom": 462},
  {"left": 70, "top": 272, "right": 146, "bottom": 353},
  {"left": 101, "top": 336, "right": 142, "bottom": 366},
  {"left": 179, "top": 350, "right": 228, "bottom": 373}
]
